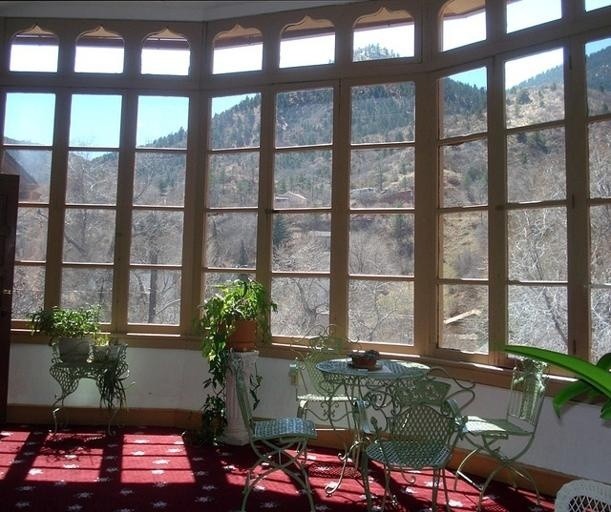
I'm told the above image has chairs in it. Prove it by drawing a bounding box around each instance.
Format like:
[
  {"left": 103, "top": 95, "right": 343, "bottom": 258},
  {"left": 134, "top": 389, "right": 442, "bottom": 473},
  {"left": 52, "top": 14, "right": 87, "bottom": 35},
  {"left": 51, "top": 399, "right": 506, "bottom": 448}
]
[
  {"left": 554, "top": 479, "right": 611, "bottom": 512},
  {"left": 235, "top": 366, "right": 319, "bottom": 511},
  {"left": 453, "top": 357, "right": 549, "bottom": 505},
  {"left": 359, "top": 366, "right": 477, "bottom": 512},
  {"left": 288, "top": 323, "right": 368, "bottom": 470}
]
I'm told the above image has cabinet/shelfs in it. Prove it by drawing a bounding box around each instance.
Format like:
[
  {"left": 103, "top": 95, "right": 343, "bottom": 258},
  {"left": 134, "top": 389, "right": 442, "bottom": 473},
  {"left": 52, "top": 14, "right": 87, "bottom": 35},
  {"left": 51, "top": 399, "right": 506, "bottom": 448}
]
[{"left": 48, "top": 344, "right": 137, "bottom": 437}]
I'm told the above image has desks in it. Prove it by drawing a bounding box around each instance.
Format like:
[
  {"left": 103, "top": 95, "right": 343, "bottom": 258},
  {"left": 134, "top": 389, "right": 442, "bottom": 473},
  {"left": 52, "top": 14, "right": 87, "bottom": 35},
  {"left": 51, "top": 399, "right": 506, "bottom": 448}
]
[{"left": 316, "top": 358, "right": 432, "bottom": 508}]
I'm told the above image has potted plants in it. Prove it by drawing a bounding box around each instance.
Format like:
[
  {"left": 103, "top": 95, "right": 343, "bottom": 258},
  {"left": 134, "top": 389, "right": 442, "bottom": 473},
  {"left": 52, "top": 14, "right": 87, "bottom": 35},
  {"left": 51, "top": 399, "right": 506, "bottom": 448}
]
[
  {"left": 181, "top": 273, "right": 278, "bottom": 449},
  {"left": 25, "top": 303, "right": 104, "bottom": 366},
  {"left": 91, "top": 332, "right": 121, "bottom": 368}
]
